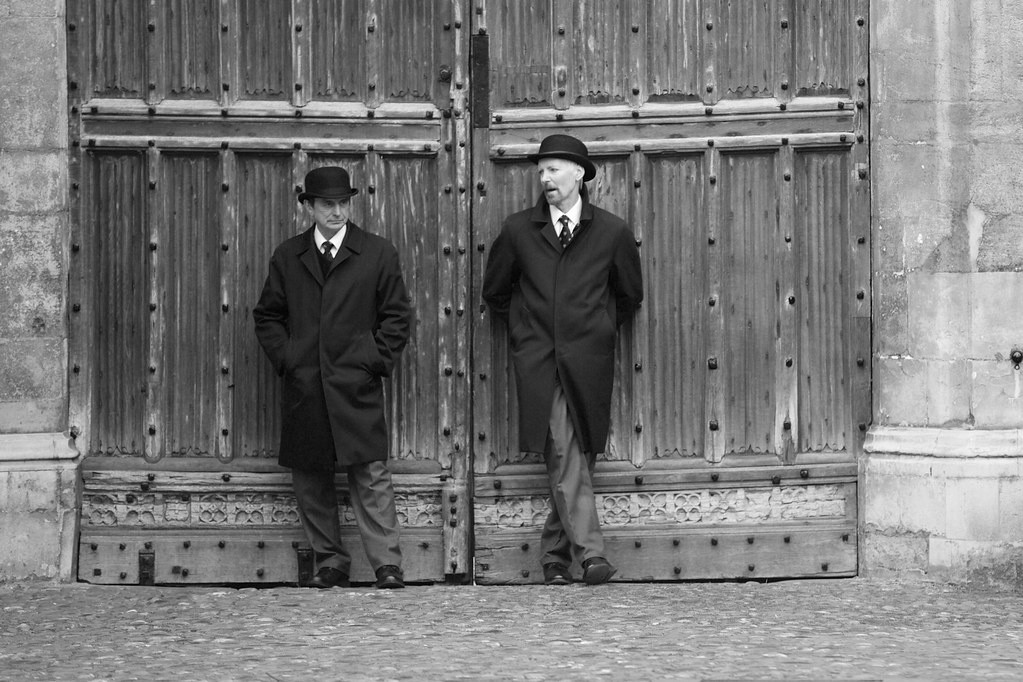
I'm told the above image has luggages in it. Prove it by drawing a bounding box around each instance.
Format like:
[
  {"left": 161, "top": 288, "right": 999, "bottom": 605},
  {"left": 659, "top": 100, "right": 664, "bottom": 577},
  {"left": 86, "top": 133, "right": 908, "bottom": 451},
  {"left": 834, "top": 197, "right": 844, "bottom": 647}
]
[{"left": 375, "top": 566, "right": 407, "bottom": 588}]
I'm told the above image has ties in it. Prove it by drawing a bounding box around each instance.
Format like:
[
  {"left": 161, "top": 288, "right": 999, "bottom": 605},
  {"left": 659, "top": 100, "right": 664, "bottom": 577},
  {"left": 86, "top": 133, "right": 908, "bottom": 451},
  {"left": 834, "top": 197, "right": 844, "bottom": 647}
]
[
  {"left": 320, "top": 242, "right": 336, "bottom": 262},
  {"left": 557, "top": 215, "right": 573, "bottom": 248}
]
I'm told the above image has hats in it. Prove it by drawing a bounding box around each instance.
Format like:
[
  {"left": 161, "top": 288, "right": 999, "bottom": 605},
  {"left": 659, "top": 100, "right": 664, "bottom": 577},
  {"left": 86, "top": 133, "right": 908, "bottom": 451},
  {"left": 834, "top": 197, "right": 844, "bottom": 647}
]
[{"left": 298, "top": 167, "right": 360, "bottom": 207}]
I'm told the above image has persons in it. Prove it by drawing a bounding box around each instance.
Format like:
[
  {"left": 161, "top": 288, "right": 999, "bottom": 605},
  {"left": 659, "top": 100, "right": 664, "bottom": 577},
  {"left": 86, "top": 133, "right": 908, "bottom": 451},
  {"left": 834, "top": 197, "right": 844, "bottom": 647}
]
[
  {"left": 483, "top": 134, "right": 644, "bottom": 587},
  {"left": 253, "top": 166, "right": 411, "bottom": 588}
]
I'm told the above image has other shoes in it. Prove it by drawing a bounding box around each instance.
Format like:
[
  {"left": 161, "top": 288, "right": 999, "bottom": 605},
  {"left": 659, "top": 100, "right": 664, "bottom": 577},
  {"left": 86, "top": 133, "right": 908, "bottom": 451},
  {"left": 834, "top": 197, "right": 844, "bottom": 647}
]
[
  {"left": 309, "top": 566, "right": 348, "bottom": 588},
  {"left": 541, "top": 562, "right": 574, "bottom": 584},
  {"left": 581, "top": 556, "right": 617, "bottom": 586},
  {"left": 527, "top": 135, "right": 596, "bottom": 182}
]
